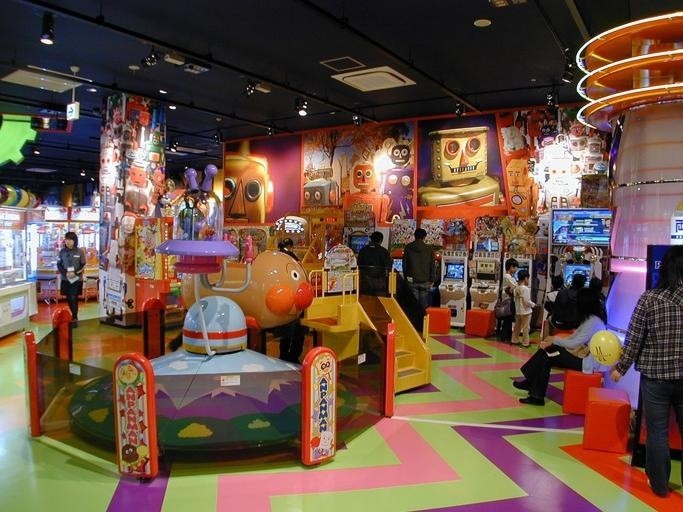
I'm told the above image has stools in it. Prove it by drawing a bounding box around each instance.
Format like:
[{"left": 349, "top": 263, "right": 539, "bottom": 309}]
[
  {"left": 563, "top": 369, "right": 602, "bottom": 415},
  {"left": 550, "top": 326, "right": 576, "bottom": 338},
  {"left": 582, "top": 387, "right": 631, "bottom": 454},
  {"left": 425, "top": 307, "right": 451, "bottom": 334},
  {"left": 465, "top": 308, "right": 496, "bottom": 337}
]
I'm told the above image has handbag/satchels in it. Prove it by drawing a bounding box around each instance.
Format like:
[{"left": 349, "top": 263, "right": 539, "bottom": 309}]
[
  {"left": 495, "top": 300, "right": 515, "bottom": 318},
  {"left": 562, "top": 344, "right": 590, "bottom": 358}
]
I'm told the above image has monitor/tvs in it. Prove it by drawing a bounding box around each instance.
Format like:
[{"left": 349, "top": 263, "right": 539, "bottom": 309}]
[
  {"left": 446, "top": 264, "right": 464, "bottom": 279},
  {"left": 552, "top": 210, "right": 612, "bottom": 246},
  {"left": 348, "top": 236, "right": 368, "bottom": 253},
  {"left": 565, "top": 264, "right": 590, "bottom": 288},
  {"left": 513, "top": 267, "right": 527, "bottom": 283},
  {"left": 393, "top": 259, "right": 404, "bottom": 272}
]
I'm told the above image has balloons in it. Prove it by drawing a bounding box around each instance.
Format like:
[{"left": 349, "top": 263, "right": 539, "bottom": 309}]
[{"left": 588, "top": 330, "right": 624, "bottom": 365}]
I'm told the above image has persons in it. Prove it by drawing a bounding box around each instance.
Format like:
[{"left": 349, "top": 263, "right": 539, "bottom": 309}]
[
  {"left": 278, "top": 238, "right": 299, "bottom": 264},
  {"left": 611, "top": 245, "right": 683, "bottom": 498},
  {"left": 58, "top": 232, "right": 87, "bottom": 323},
  {"left": 401, "top": 227, "right": 434, "bottom": 322},
  {"left": 357, "top": 231, "right": 394, "bottom": 272},
  {"left": 499, "top": 257, "right": 607, "bottom": 406}
]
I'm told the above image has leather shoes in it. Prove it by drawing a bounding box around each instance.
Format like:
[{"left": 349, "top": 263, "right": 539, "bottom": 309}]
[
  {"left": 519, "top": 396, "right": 545, "bottom": 406},
  {"left": 513, "top": 380, "right": 531, "bottom": 391}
]
